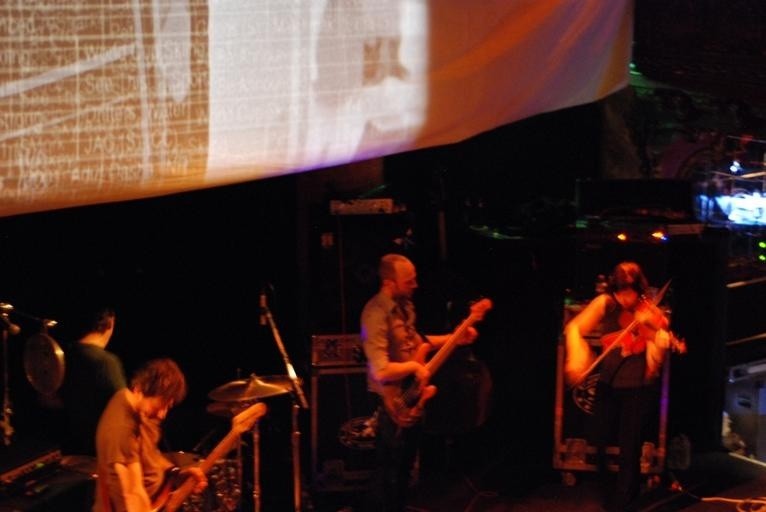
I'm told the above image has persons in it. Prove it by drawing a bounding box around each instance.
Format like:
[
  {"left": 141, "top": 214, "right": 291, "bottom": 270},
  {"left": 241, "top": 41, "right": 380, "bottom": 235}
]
[
  {"left": 360, "top": 253, "right": 479, "bottom": 510},
  {"left": 61, "top": 304, "right": 130, "bottom": 456},
  {"left": 565, "top": 260, "right": 671, "bottom": 510},
  {"left": 92, "top": 357, "right": 210, "bottom": 512}
]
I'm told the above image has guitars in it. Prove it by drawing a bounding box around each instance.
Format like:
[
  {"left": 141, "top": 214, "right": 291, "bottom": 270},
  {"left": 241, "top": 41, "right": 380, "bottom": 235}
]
[
  {"left": 380, "top": 299, "right": 492, "bottom": 427},
  {"left": 149, "top": 402, "right": 267, "bottom": 511}
]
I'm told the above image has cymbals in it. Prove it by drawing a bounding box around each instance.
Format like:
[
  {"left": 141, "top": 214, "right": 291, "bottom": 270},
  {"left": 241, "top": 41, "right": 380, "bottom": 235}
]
[{"left": 209, "top": 376, "right": 302, "bottom": 401}]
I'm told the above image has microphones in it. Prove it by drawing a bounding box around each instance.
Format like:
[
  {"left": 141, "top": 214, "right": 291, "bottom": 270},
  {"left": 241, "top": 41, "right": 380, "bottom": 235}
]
[{"left": 259, "top": 295, "right": 267, "bottom": 325}]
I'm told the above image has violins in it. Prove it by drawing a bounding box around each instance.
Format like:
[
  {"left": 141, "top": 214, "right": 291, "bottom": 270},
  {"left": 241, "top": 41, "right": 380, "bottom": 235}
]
[{"left": 621, "top": 299, "right": 686, "bottom": 353}]
